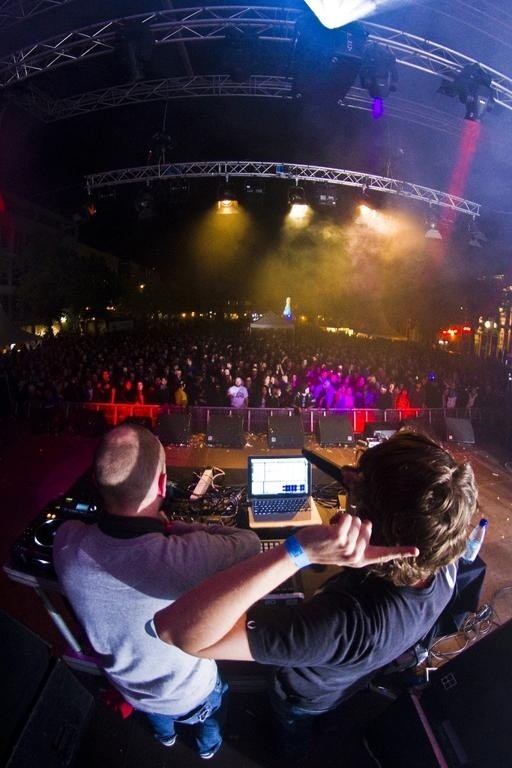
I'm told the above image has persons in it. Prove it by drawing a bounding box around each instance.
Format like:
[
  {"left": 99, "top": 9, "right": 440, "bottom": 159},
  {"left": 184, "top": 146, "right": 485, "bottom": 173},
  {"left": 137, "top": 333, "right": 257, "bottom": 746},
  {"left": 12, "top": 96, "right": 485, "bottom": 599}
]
[
  {"left": 0, "top": 313, "right": 511, "bottom": 415},
  {"left": 50, "top": 419, "right": 265, "bottom": 759},
  {"left": 147, "top": 419, "right": 483, "bottom": 766}
]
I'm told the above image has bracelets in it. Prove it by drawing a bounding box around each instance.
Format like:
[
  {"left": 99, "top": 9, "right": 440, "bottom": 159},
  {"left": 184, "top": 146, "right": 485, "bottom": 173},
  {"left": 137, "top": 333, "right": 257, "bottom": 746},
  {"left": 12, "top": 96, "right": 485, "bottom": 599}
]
[{"left": 282, "top": 536, "right": 311, "bottom": 570}]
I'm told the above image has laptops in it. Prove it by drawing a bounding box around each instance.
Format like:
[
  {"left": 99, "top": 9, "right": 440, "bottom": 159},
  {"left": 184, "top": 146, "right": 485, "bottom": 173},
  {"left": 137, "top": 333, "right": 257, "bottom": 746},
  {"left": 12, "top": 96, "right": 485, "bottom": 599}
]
[{"left": 246, "top": 454, "right": 321, "bottom": 532}]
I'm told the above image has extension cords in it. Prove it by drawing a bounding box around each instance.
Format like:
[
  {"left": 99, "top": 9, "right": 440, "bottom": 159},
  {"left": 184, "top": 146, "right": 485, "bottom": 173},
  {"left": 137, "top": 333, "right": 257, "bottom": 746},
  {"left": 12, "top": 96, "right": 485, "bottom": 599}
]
[{"left": 188, "top": 468, "right": 214, "bottom": 500}]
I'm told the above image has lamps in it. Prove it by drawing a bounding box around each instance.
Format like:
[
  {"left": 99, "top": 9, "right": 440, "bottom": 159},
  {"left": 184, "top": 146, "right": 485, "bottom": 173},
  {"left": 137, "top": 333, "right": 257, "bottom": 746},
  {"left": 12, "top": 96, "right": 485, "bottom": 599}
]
[
  {"left": 216, "top": 16, "right": 264, "bottom": 84},
  {"left": 218, "top": 172, "right": 483, "bottom": 240},
  {"left": 437, "top": 52, "right": 497, "bottom": 124},
  {"left": 362, "top": 39, "right": 400, "bottom": 104}
]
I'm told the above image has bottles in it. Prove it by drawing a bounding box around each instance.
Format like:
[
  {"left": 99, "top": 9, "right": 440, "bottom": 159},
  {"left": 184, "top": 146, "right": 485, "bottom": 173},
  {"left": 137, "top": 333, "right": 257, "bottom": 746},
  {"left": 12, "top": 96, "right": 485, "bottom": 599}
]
[{"left": 462, "top": 519, "right": 488, "bottom": 562}]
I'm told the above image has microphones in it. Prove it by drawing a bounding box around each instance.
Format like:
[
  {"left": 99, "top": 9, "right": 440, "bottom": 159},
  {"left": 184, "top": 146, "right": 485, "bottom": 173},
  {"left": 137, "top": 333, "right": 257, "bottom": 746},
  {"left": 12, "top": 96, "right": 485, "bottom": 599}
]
[{"left": 301, "top": 447, "right": 352, "bottom": 489}]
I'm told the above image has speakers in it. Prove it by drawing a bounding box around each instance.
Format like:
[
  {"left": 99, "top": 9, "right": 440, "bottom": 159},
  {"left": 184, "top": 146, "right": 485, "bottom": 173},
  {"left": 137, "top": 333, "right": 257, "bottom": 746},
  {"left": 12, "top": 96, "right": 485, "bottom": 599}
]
[
  {"left": 206, "top": 414, "right": 244, "bottom": 449},
  {"left": 316, "top": 416, "right": 354, "bottom": 448},
  {"left": 440, "top": 416, "right": 476, "bottom": 446},
  {"left": 266, "top": 415, "right": 303, "bottom": 450},
  {"left": 156, "top": 414, "right": 191, "bottom": 448}
]
